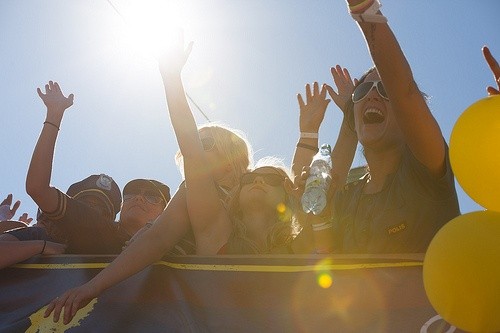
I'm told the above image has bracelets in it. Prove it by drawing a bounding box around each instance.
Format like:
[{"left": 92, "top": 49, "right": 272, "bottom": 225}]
[
  {"left": 44, "top": 121, "right": 60, "bottom": 131},
  {"left": 295, "top": 142, "right": 318, "bottom": 152},
  {"left": 299, "top": 131, "right": 318, "bottom": 138},
  {"left": 40, "top": 238, "right": 47, "bottom": 255},
  {"left": 348, "top": 0, "right": 388, "bottom": 22}
]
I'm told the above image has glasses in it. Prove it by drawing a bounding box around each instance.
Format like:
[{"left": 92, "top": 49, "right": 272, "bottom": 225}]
[
  {"left": 201, "top": 136, "right": 220, "bottom": 151},
  {"left": 352, "top": 80, "right": 392, "bottom": 103},
  {"left": 123, "top": 189, "right": 165, "bottom": 204},
  {"left": 240, "top": 172, "right": 291, "bottom": 189}
]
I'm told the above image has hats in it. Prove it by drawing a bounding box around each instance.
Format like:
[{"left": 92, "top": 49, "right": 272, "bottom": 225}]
[
  {"left": 123, "top": 179, "right": 171, "bottom": 206},
  {"left": 65, "top": 174, "right": 122, "bottom": 221}
]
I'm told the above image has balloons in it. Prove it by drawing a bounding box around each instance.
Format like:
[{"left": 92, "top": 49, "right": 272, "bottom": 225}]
[
  {"left": 448, "top": 94, "right": 500, "bottom": 211},
  {"left": 422, "top": 210, "right": 499, "bottom": 332}
]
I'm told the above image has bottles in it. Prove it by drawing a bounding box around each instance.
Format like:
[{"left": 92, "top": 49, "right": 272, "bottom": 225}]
[{"left": 301, "top": 144, "right": 334, "bottom": 216}]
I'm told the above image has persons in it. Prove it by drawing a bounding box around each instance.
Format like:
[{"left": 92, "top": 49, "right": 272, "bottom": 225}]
[{"left": 0, "top": 0, "right": 500, "bottom": 333}]
